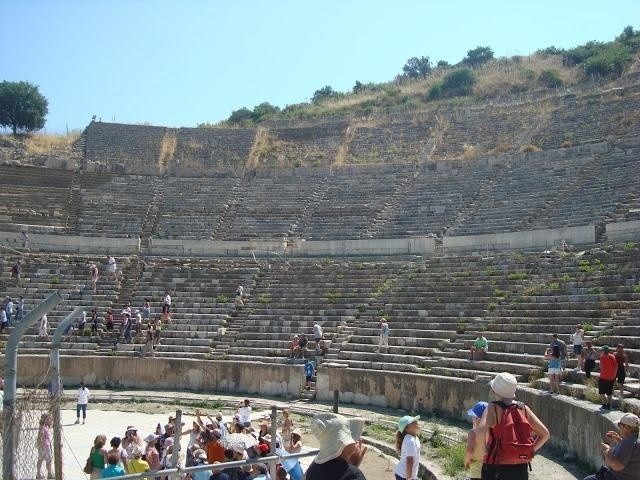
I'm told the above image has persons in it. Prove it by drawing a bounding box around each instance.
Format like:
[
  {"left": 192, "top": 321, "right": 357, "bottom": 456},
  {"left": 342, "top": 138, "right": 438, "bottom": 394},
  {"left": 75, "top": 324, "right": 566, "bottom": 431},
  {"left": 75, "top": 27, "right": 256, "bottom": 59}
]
[
  {"left": 235, "top": 283, "right": 244, "bottom": 307},
  {"left": 8, "top": 261, "right": 20, "bottom": 281},
  {"left": 75, "top": 382, "right": 89, "bottom": 424},
  {"left": 82, "top": 398, "right": 306, "bottom": 480},
  {"left": 469, "top": 332, "right": 489, "bottom": 360},
  {"left": 464, "top": 373, "right": 549, "bottom": 480},
  {"left": 34, "top": 413, "right": 54, "bottom": 478},
  {"left": 375, "top": 318, "right": 391, "bottom": 354},
  {"left": 305, "top": 412, "right": 368, "bottom": 479},
  {"left": 106, "top": 255, "right": 118, "bottom": 282},
  {"left": 88, "top": 309, "right": 100, "bottom": 336},
  {"left": 77, "top": 310, "right": 86, "bottom": 336},
  {"left": 291, "top": 321, "right": 322, "bottom": 391},
  {"left": 88, "top": 263, "right": 98, "bottom": 292},
  {"left": 120, "top": 299, "right": 161, "bottom": 359},
  {"left": 394, "top": 414, "right": 422, "bottom": 480},
  {"left": 38, "top": 315, "right": 51, "bottom": 342},
  {"left": 162, "top": 292, "right": 171, "bottom": 319},
  {"left": 544, "top": 326, "right": 630, "bottom": 411},
  {"left": 105, "top": 310, "right": 114, "bottom": 335},
  {"left": 1, "top": 295, "right": 24, "bottom": 327},
  {"left": 594, "top": 413, "right": 639, "bottom": 479}
]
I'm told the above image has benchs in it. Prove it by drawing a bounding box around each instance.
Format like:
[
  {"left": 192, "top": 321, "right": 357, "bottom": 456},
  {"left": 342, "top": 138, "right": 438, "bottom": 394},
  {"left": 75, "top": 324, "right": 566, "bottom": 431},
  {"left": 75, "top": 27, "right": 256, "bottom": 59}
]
[
  {"left": 315, "top": 87, "right": 639, "bottom": 418},
  {"left": 2, "top": 119, "right": 315, "bottom": 401}
]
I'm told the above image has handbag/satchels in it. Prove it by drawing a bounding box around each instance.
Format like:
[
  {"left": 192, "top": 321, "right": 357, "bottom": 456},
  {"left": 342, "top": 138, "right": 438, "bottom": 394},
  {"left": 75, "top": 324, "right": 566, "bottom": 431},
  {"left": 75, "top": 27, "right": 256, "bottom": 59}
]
[{"left": 84, "top": 458, "right": 92, "bottom": 474}]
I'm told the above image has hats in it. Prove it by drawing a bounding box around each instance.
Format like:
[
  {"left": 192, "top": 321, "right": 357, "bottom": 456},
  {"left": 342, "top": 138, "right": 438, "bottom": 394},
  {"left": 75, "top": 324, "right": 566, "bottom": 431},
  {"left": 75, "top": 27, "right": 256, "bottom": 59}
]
[
  {"left": 187, "top": 412, "right": 301, "bottom": 466},
  {"left": 467, "top": 401, "right": 489, "bottom": 418},
  {"left": 398, "top": 415, "right": 421, "bottom": 434},
  {"left": 126, "top": 415, "right": 176, "bottom": 457},
  {"left": 311, "top": 412, "right": 366, "bottom": 465},
  {"left": 619, "top": 413, "right": 640, "bottom": 427},
  {"left": 490, "top": 371, "right": 519, "bottom": 399}
]
